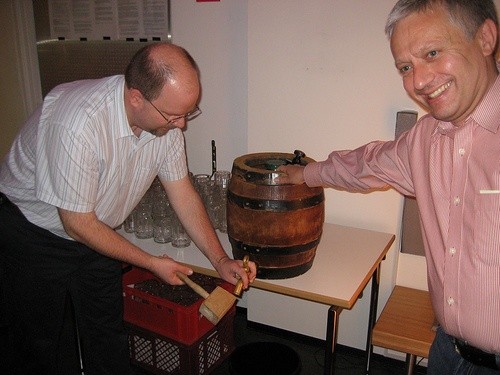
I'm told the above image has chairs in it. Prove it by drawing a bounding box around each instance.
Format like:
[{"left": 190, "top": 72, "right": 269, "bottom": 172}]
[{"left": 371, "top": 196, "right": 436, "bottom": 375}]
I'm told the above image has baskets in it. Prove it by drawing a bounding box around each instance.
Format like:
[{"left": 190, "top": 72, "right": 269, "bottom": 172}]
[{"left": 122, "top": 267, "right": 236, "bottom": 375}]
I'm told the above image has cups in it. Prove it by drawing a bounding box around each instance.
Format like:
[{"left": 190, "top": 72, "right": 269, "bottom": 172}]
[{"left": 124, "top": 171, "right": 230, "bottom": 248}]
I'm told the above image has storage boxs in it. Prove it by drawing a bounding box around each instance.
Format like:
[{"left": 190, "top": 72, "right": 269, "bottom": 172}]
[{"left": 122, "top": 267, "right": 238, "bottom": 375}]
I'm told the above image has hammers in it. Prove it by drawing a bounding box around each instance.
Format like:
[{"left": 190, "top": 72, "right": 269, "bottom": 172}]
[{"left": 163, "top": 256, "right": 237, "bottom": 326}]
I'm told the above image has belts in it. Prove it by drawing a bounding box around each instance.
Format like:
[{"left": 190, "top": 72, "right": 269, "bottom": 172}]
[{"left": 449, "top": 336, "right": 500, "bottom": 371}]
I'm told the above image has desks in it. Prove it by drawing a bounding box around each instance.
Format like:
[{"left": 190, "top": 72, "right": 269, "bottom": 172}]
[{"left": 74, "top": 218, "right": 395, "bottom": 375}]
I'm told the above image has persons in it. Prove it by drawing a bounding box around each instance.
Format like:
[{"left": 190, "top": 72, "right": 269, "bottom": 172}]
[
  {"left": 0, "top": 43, "right": 256, "bottom": 375},
  {"left": 274, "top": 0, "right": 500, "bottom": 375}
]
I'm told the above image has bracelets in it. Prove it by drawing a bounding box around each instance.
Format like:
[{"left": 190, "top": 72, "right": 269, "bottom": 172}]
[{"left": 210, "top": 254, "right": 229, "bottom": 268}]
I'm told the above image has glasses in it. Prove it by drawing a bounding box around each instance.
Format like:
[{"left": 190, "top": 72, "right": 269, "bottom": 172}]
[{"left": 133, "top": 88, "right": 199, "bottom": 127}]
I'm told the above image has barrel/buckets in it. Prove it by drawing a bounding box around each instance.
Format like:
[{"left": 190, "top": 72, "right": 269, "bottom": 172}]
[
  {"left": 226, "top": 152, "right": 326, "bottom": 280},
  {"left": 228, "top": 341, "right": 301, "bottom": 375}
]
